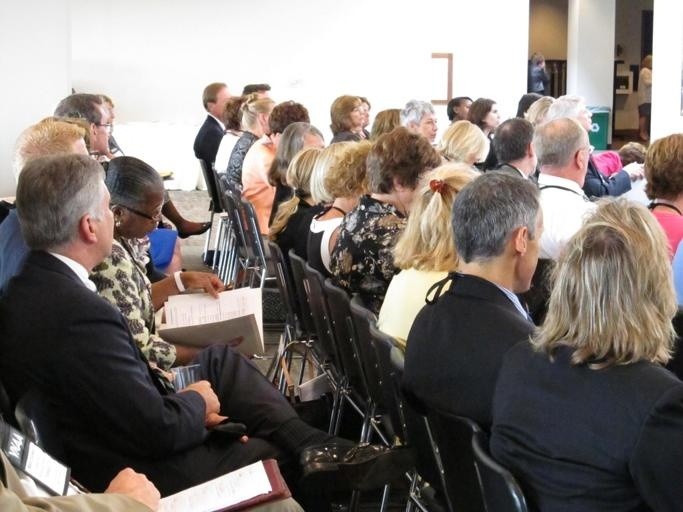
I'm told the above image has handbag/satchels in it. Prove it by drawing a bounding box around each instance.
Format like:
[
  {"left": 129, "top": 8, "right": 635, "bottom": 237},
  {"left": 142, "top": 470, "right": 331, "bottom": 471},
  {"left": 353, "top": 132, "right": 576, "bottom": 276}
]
[{"left": 280, "top": 340, "right": 359, "bottom": 441}]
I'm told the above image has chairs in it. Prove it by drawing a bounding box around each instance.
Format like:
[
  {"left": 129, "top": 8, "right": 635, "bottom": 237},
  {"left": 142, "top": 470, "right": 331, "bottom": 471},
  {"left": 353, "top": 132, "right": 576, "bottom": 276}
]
[{"left": 197, "top": 160, "right": 533, "bottom": 512}]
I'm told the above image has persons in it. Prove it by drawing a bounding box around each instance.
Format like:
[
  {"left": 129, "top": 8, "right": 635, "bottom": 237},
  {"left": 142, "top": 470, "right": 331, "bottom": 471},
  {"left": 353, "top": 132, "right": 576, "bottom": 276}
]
[
  {"left": 529, "top": 52, "right": 550, "bottom": 95},
  {"left": 0, "top": 82, "right": 682, "bottom": 511},
  {"left": 637, "top": 55, "right": 652, "bottom": 142}
]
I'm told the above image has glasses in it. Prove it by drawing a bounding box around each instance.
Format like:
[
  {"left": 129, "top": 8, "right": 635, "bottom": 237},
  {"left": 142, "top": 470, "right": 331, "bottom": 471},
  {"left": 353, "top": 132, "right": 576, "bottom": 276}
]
[
  {"left": 586, "top": 145, "right": 595, "bottom": 154},
  {"left": 96, "top": 124, "right": 112, "bottom": 133},
  {"left": 118, "top": 205, "right": 162, "bottom": 222}
]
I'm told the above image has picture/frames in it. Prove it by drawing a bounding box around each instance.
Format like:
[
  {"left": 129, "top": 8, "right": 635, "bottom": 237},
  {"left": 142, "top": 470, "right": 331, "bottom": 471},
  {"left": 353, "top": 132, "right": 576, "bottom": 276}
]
[{"left": 430, "top": 54, "right": 452, "bottom": 104}]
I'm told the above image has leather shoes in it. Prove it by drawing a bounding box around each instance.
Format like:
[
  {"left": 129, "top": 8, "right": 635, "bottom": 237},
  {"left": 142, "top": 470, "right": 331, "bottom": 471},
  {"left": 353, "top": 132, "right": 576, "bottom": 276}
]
[
  {"left": 298, "top": 441, "right": 418, "bottom": 492},
  {"left": 177, "top": 222, "right": 210, "bottom": 238}
]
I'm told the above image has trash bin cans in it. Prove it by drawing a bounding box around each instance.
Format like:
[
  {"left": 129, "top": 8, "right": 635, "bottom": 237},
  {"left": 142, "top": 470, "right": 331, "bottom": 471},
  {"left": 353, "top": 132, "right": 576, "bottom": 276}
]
[{"left": 588, "top": 108, "right": 608, "bottom": 150}]
